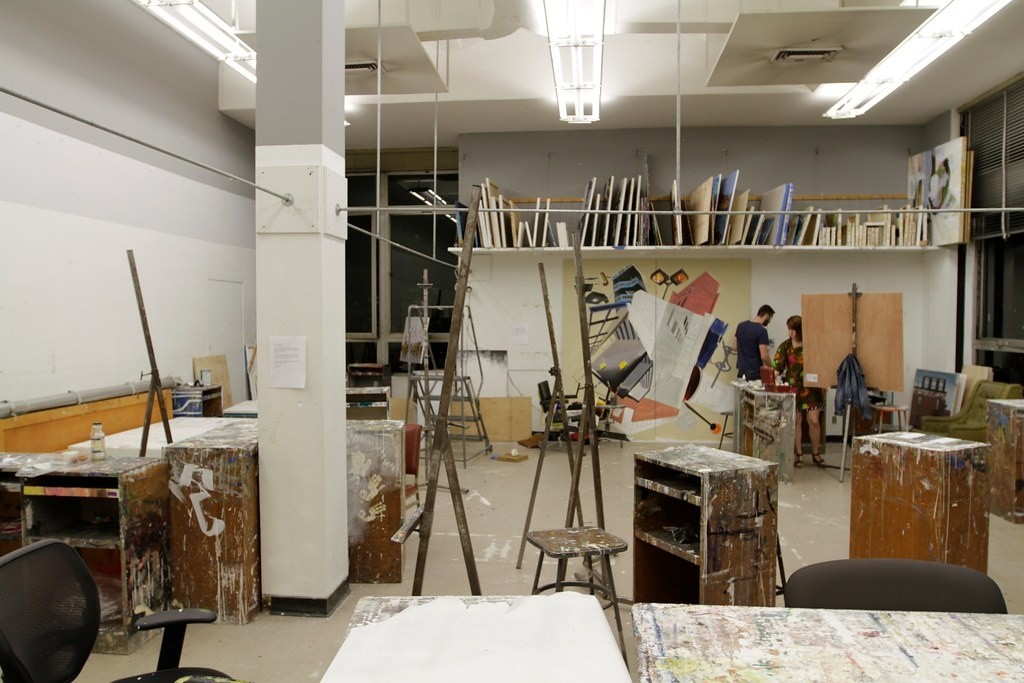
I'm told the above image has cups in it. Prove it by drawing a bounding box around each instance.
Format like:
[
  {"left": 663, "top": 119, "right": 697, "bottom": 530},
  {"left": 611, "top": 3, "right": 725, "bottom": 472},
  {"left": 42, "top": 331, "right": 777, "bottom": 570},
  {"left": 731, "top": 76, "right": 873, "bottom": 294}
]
[{"left": 62, "top": 451, "right": 79, "bottom": 461}]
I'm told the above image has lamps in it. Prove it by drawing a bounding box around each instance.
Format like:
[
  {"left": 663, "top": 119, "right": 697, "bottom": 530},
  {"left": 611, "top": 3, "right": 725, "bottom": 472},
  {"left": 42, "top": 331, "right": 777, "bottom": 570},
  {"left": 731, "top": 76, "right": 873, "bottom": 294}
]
[
  {"left": 126, "top": 0, "right": 258, "bottom": 84},
  {"left": 542, "top": 0, "right": 606, "bottom": 124},
  {"left": 572, "top": 266, "right": 689, "bottom": 305},
  {"left": 823, "top": 0, "right": 1014, "bottom": 120}
]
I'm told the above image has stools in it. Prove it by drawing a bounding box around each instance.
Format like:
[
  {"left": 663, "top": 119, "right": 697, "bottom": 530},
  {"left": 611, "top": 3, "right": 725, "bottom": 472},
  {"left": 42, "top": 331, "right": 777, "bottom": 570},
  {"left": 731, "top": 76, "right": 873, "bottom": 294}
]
[
  {"left": 870, "top": 404, "right": 908, "bottom": 435},
  {"left": 524, "top": 529, "right": 629, "bottom": 672}
]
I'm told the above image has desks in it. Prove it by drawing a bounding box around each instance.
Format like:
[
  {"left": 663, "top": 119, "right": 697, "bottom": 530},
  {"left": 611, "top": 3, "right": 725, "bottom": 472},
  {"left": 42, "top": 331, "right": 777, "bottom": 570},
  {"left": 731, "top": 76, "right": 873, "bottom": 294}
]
[
  {"left": 324, "top": 597, "right": 1024, "bottom": 683},
  {"left": 223, "top": 400, "right": 258, "bottom": 419},
  {"left": 160, "top": 416, "right": 259, "bottom": 622},
  {"left": 68, "top": 416, "right": 237, "bottom": 458}
]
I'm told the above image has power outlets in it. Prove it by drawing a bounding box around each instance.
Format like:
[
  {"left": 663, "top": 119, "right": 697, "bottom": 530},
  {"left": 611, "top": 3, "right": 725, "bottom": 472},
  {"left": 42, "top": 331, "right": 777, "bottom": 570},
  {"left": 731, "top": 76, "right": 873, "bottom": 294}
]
[{"left": 832, "top": 416, "right": 836, "bottom": 424}]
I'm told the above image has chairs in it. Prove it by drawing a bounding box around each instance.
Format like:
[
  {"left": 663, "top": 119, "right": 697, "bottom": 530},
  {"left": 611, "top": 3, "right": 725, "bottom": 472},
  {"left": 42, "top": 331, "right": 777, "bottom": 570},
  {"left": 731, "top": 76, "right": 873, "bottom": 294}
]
[
  {"left": 919, "top": 379, "right": 1024, "bottom": 443},
  {"left": 538, "top": 380, "right": 577, "bottom": 450},
  {"left": 781, "top": 556, "right": 1008, "bottom": 612},
  {"left": 0, "top": 538, "right": 234, "bottom": 683}
]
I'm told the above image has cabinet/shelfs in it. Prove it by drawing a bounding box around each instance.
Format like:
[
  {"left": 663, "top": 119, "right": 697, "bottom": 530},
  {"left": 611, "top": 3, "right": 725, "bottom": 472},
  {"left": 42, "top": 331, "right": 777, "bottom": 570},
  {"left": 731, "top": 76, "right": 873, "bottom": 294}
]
[
  {"left": 172, "top": 384, "right": 223, "bottom": 418},
  {"left": 987, "top": 397, "right": 1024, "bottom": 524},
  {"left": 730, "top": 380, "right": 796, "bottom": 481},
  {"left": 633, "top": 444, "right": 779, "bottom": 609},
  {"left": 413, "top": 369, "right": 450, "bottom": 449},
  {"left": 851, "top": 429, "right": 993, "bottom": 582},
  {"left": 345, "top": 418, "right": 406, "bottom": 584},
  {"left": 346, "top": 386, "right": 390, "bottom": 420},
  {"left": 0, "top": 451, "right": 168, "bottom": 656},
  {"left": 348, "top": 363, "right": 383, "bottom": 386}
]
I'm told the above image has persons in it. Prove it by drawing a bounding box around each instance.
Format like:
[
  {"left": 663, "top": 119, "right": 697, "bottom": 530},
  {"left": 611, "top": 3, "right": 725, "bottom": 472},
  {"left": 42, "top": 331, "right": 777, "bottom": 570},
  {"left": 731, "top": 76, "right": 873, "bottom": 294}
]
[
  {"left": 772, "top": 315, "right": 826, "bottom": 467},
  {"left": 734, "top": 304, "right": 775, "bottom": 382}
]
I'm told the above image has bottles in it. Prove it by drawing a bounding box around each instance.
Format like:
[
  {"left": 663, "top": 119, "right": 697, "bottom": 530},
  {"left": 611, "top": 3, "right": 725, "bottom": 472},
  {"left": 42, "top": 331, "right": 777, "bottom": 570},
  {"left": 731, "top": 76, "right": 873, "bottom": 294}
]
[{"left": 90, "top": 421, "right": 106, "bottom": 463}]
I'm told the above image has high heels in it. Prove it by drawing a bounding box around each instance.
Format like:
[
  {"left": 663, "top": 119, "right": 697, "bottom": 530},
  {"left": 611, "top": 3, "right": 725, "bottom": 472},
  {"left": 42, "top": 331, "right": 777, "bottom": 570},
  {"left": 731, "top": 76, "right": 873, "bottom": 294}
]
[
  {"left": 812, "top": 453, "right": 827, "bottom": 467},
  {"left": 794, "top": 453, "right": 804, "bottom": 468}
]
